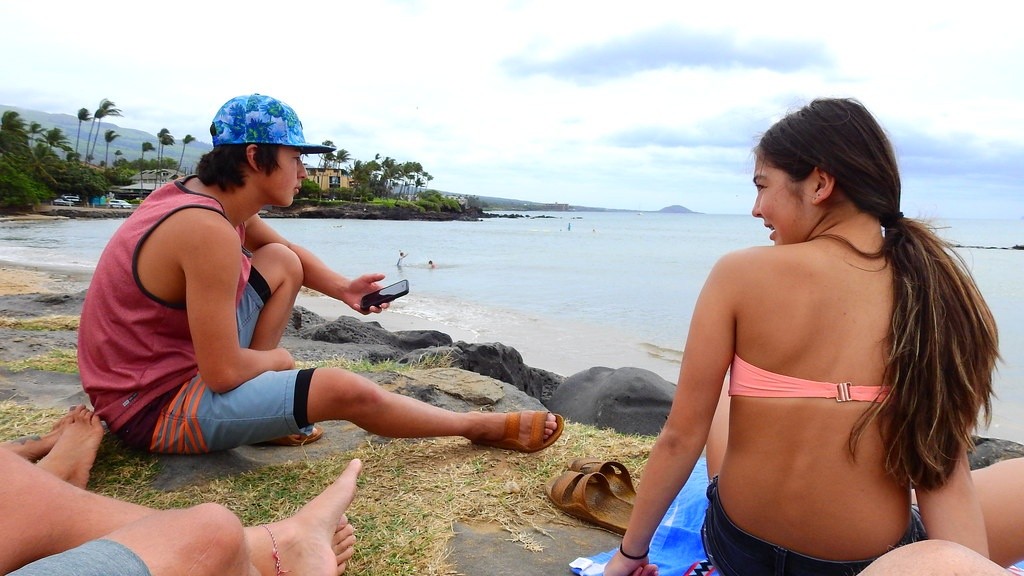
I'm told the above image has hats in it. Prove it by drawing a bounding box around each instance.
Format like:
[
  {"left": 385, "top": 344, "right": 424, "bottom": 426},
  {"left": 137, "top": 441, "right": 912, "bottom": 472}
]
[{"left": 210, "top": 93, "right": 337, "bottom": 154}]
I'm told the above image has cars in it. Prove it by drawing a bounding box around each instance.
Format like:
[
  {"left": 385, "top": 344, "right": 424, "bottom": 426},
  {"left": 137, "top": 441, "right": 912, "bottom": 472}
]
[{"left": 53, "top": 194, "right": 80, "bottom": 207}]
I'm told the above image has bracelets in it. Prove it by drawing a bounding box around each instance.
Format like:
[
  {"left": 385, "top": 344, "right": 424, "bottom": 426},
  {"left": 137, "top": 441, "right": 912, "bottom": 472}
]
[{"left": 620, "top": 542, "right": 650, "bottom": 560}]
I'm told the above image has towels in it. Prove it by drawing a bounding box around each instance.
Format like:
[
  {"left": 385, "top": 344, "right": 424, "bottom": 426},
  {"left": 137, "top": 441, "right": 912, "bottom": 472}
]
[{"left": 568, "top": 455, "right": 1024, "bottom": 575}]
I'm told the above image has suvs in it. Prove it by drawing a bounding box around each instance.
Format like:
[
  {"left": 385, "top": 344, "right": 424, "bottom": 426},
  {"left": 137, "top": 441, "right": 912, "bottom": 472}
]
[{"left": 107, "top": 200, "right": 132, "bottom": 208}]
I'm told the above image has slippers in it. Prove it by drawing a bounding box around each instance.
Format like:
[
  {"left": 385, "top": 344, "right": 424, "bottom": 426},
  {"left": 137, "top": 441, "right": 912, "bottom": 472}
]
[
  {"left": 467, "top": 409, "right": 565, "bottom": 452},
  {"left": 256, "top": 423, "right": 326, "bottom": 446},
  {"left": 545, "top": 470, "right": 634, "bottom": 537},
  {"left": 565, "top": 457, "right": 642, "bottom": 506}
]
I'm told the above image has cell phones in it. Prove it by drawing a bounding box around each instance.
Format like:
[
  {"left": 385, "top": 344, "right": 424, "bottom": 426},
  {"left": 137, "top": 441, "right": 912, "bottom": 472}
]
[{"left": 361, "top": 279, "right": 409, "bottom": 310}]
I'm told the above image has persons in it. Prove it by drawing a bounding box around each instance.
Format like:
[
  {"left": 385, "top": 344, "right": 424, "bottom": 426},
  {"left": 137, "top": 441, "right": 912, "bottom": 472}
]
[
  {"left": 397, "top": 250, "right": 408, "bottom": 265},
  {"left": 0, "top": 406, "right": 362, "bottom": 576},
  {"left": 855, "top": 539, "right": 1018, "bottom": 576},
  {"left": 568, "top": 223, "right": 570, "bottom": 231},
  {"left": 78, "top": 93, "right": 565, "bottom": 455},
  {"left": 429, "top": 261, "right": 435, "bottom": 268},
  {"left": 602, "top": 98, "right": 1024, "bottom": 576}
]
